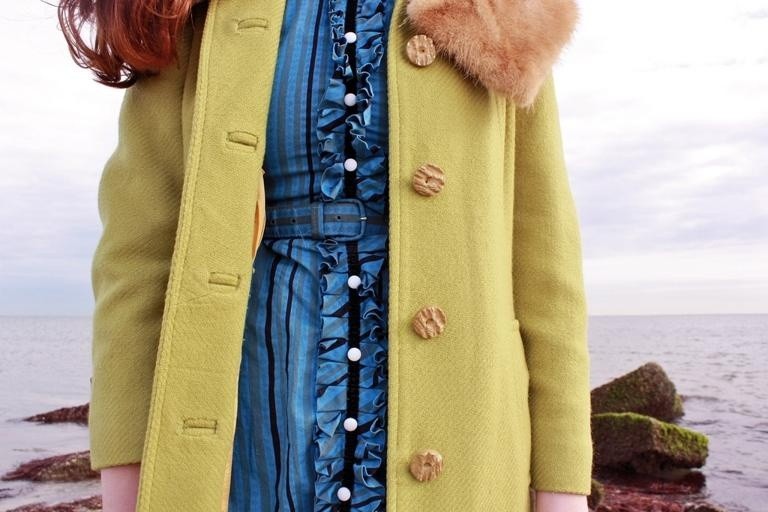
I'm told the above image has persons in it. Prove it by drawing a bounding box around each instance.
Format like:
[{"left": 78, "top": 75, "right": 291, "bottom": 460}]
[{"left": 54, "top": 1, "right": 593, "bottom": 512}]
